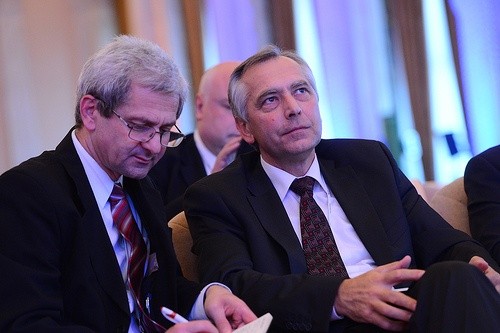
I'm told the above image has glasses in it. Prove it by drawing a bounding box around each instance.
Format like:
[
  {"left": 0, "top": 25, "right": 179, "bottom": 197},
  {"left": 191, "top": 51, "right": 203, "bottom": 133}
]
[{"left": 113, "top": 108, "right": 184, "bottom": 148}]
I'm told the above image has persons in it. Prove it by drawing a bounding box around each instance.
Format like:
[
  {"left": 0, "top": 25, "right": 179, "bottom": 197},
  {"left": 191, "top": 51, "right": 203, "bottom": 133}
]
[
  {"left": 0, "top": 37, "right": 257, "bottom": 333},
  {"left": 148, "top": 60, "right": 243, "bottom": 221},
  {"left": 183, "top": 48, "right": 500, "bottom": 333}
]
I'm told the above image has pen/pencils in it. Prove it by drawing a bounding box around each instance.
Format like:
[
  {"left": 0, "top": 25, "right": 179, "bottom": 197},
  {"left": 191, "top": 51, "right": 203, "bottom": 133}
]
[{"left": 161, "top": 306, "right": 189, "bottom": 326}]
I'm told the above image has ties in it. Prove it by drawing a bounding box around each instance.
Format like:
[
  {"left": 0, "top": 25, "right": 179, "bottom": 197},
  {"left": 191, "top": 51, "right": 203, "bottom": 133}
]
[
  {"left": 108, "top": 182, "right": 168, "bottom": 333},
  {"left": 289, "top": 177, "right": 351, "bottom": 283}
]
